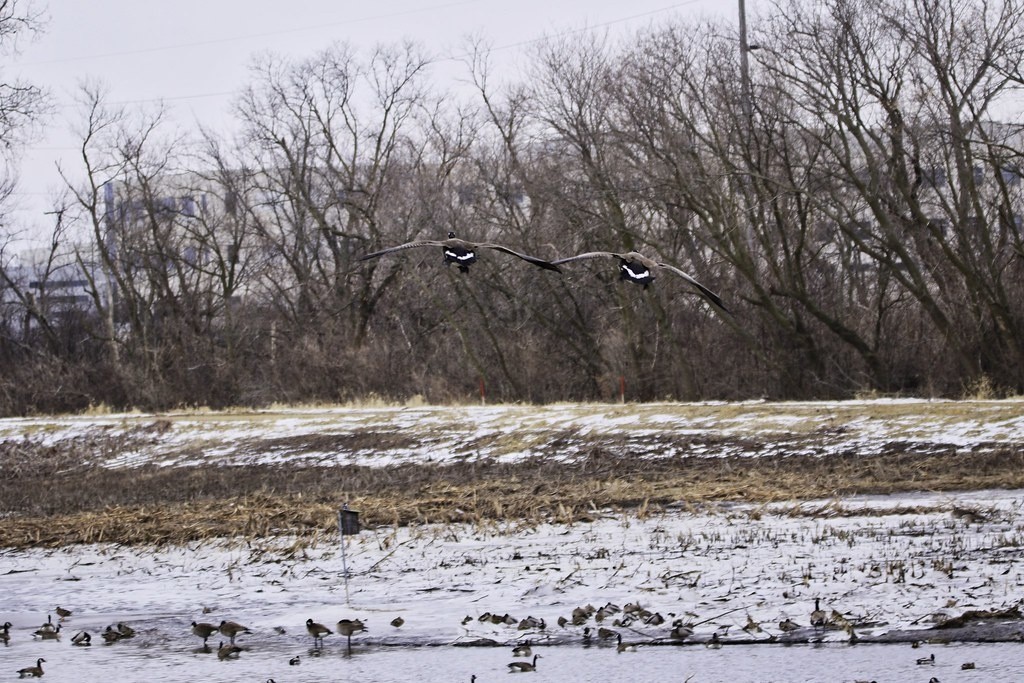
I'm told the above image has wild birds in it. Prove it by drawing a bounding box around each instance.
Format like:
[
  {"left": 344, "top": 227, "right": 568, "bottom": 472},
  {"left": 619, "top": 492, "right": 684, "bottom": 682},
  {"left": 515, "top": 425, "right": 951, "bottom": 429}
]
[
  {"left": 357, "top": 230, "right": 565, "bottom": 276},
  {"left": 535, "top": 248, "right": 734, "bottom": 316},
  {"left": 0, "top": 594, "right": 1024, "bottom": 683}
]
